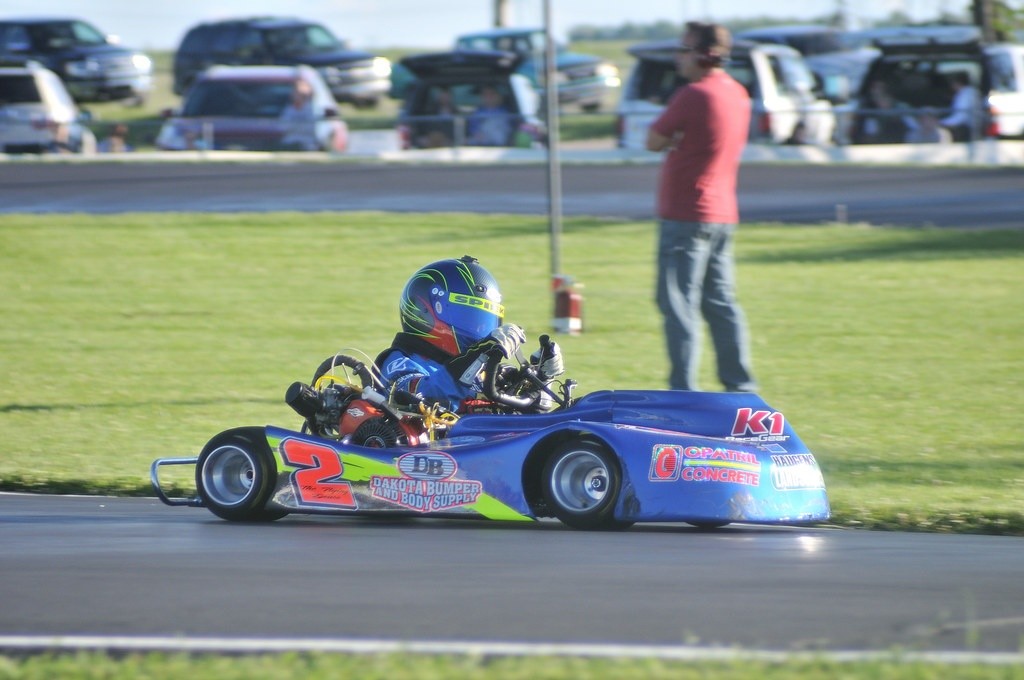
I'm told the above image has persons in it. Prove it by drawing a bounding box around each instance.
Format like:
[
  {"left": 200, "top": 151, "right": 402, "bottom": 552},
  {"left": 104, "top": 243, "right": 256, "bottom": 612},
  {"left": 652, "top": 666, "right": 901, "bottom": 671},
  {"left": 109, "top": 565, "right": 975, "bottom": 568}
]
[
  {"left": 413, "top": 88, "right": 512, "bottom": 147},
  {"left": 375, "top": 258, "right": 564, "bottom": 443},
  {"left": 939, "top": 72, "right": 983, "bottom": 143},
  {"left": 848, "top": 74, "right": 921, "bottom": 143},
  {"left": 645, "top": 23, "right": 760, "bottom": 393}
]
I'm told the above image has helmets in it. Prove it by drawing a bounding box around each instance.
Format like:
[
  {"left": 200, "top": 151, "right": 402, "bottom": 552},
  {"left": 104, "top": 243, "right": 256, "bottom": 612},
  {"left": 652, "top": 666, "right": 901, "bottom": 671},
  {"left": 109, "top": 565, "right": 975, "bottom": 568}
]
[{"left": 399, "top": 255, "right": 505, "bottom": 355}]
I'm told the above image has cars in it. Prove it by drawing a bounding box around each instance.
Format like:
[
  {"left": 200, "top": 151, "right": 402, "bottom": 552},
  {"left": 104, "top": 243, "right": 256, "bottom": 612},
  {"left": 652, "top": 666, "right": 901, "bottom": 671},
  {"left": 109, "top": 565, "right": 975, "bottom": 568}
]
[
  {"left": 157, "top": 63, "right": 350, "bottom": 151},
  {"left": 614, "top": 40, "right": 837, "bottom": 145},
  {"left": 849, "top": 31, "right": 1024, "bottom": 144},
  {"left": 0, "top": 19, "right": 154, "bottom": 111},
  {"left": 741, "top": 25, "right": 886, "bottom": 137},
  {"left": 173, "top": 18, "right": 390, "bottom": 114},
  {"left": 399, "top": 52, "right": 543, "bottom": 148},
  {"left": 1, "top": 57, "right": 99, "bottom": 156}
]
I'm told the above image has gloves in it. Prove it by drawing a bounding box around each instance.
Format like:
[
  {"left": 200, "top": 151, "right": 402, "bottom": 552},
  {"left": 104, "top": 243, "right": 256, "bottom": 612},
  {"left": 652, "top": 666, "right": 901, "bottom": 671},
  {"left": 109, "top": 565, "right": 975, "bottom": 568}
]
[
  {"left": 448, "top": 325, "right": 526, "bottom": 384},
  {"left": 530, "top": 342, "right": 567, "bottom": 385}
]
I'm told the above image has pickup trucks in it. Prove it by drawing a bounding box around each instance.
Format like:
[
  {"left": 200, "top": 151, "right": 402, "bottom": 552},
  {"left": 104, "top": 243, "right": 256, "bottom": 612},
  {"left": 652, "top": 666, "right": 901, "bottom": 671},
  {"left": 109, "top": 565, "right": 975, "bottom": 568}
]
[{"left": 392, "top": 30, "right": 621, "bottom": 113}]
